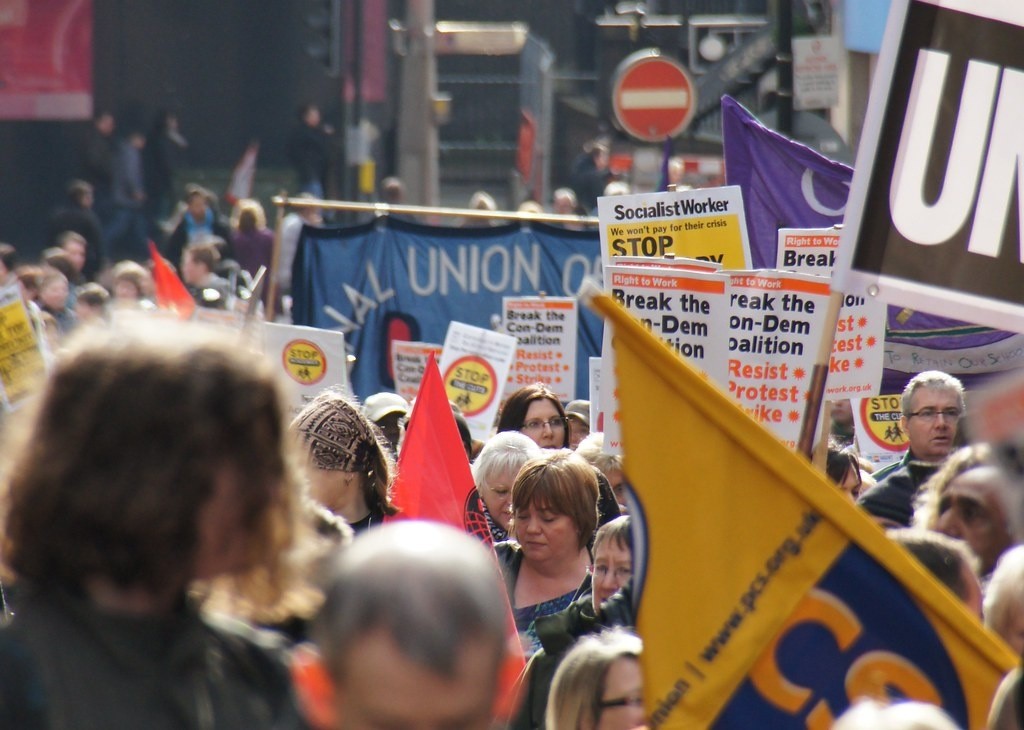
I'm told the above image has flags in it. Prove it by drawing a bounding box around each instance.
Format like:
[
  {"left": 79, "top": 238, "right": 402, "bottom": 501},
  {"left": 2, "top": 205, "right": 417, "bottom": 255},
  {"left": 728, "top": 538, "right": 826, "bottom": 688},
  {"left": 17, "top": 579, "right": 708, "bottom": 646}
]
[
  {"left": 724, "top": 94, "right": 1024, "bottom": 369},
  {"left": 298, "top": 358, "right": 529, "bottom": 730}
]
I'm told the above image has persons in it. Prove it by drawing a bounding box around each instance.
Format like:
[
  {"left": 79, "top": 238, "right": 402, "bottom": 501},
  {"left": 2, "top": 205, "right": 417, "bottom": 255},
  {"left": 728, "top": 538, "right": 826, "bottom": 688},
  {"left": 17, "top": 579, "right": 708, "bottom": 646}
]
[
  {"left": 0, "top": 181, "right": 646, "bottom": 730},
  {"left": 570, "top": 142, "right": 612, "bottom": 213},
  {"left": 291, "top": 105, "right": 334, "bottom": 203},
  {"left": 87, "top": 105, "right": 191, "bottom": 229},
  {"left": 809, "top": 370, "right": 1024, "bottom": 661}
]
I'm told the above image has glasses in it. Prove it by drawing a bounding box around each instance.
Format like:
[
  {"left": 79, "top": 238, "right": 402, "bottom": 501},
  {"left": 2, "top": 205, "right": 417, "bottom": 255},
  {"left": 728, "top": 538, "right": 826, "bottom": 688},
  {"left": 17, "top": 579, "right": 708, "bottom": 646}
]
[
  {"left": 605, "top": 688, "right": 645, "bottom": 710},
  {"left": 521, "top": 417, "right": 570, "bottom": 434},
  {"left": 586, "top": 563, "right": 632, "bottom": 579},
  {"left": 908, "top": 405, "right": 964, "bottom": 421}
]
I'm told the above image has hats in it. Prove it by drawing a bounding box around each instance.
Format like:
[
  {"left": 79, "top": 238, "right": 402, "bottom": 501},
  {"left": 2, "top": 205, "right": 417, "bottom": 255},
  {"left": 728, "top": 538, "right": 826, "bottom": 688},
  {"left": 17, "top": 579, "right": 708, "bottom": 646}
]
[
  {"left": 563, "top": 399, "right": 591, "bottom": 429},
  {"left": 361, "top": 391, "right": 408, "bottom": 426}
]
